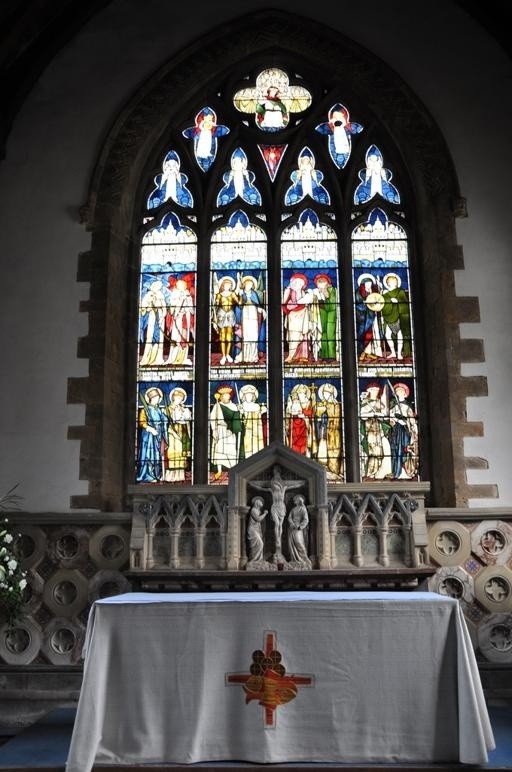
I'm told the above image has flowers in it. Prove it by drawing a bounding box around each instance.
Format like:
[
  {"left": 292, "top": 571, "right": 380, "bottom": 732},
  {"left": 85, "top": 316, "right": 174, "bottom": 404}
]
[{"left": 1, "top": 482, "right": 34, "bottom": 630}]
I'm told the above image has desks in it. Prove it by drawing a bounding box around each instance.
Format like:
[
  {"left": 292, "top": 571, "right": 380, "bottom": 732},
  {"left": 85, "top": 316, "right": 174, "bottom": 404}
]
[{"left": 64, "top": 591, "right": 499, "bottom": 770}]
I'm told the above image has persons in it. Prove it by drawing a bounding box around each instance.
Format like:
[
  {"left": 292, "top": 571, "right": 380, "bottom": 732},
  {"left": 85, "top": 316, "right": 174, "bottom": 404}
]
[
  {"left": 245, "top": 471, "right": 307, "bottom": 560},
  {"left": 288, "top": 493, "right": 310, "bottom": 565},
  {"left": 243, "top": 489, "right": 267, "bottom": 567}
]
